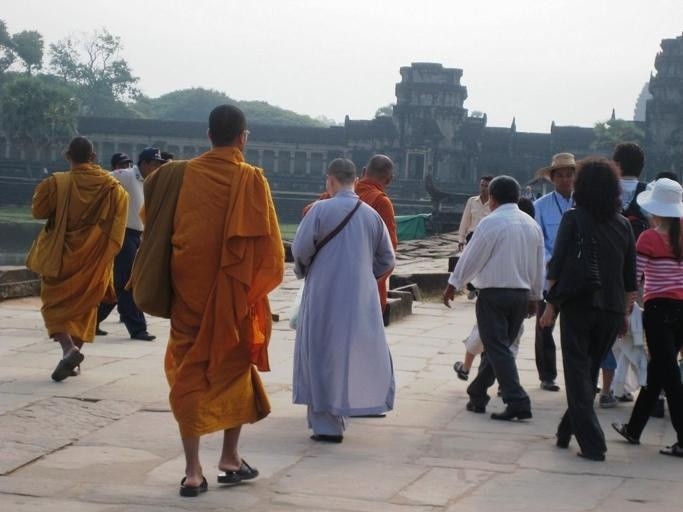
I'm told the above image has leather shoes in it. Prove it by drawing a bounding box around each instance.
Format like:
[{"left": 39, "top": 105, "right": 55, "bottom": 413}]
[
  {"left": 464, "top": 398, "right": 487, "bottom": 413},
  {"left": 489, "top": 397, "right": 533, "bottom": 422},
  {"left": 130, "top": 330, "right": 157, "bottom": 341},
  {"left": 95, "top": 322, "right": 109, "bottom": 337}
]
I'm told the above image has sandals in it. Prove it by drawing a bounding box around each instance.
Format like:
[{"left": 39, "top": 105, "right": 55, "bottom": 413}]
[
  {"left": 657, "top": 441, "right": 683, "bottom": 458},
  {"left": 609, "top": 419, "right": 641, "bottom": 446},
  {"left": 452, "top": 359, "right": 469, "bottom": 381}
]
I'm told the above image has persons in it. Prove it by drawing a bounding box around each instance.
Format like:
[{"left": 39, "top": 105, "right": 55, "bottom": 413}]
[
  {"left": 110, "top": 152, "right": 130, "bottom": 169},
  {"left": 95, "top": 147, "right": 164, "bottom": 341},
  {"left": 441, "top": 153, "right": 576, "bottom": 422},
  {"left": 143, "top": 103, "right": 284, "bottom": 496},
  {"left": 28, "top": 134, "right": 128, "bottom": 381},
  {"left": 596, "top": 142, "right": 683, "bottom": 456},
  {"left": 289, "top": 157, "right": 396, "bottom": 444},
  {"left": 540, "top": 161, "right": 639, "bottom": 462},
  {"left": 299, "top": 154, "right": 398, "bottom": 317}
]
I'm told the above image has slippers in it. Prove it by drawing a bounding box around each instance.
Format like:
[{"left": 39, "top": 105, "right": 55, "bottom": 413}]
[
  {"left": 213, "top": 457, "right": 262, "bottom": 485},
  {"left": 48, "top": 348, "right": 85, "bottom": 383},
  {"left": 177, "top": 473, "right": 209, "bottom": 500},
  {"left": 309, "top": 432, "right": 343, "bottom": 444}
]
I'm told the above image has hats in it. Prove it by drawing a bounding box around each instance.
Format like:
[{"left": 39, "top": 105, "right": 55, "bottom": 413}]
[
  {"left": 634, "top": 177, "right": 683, "bottom": 220},
  {"left": 534, "top": 152, "right": 578, "bottom": 179},
  {"left": 136, "top": 147, "right": 167, "bottom": 166},
  {"left": 109, "top": 151, "right": 133, "bottom": 167}
]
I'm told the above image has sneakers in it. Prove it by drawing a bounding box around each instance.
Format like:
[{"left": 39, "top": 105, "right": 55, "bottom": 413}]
[
  {"left": 619, "top": 388, "right": 635, "bottom": 402},
  {"left": 576, "top": 451, "right": 606, "bottom": 462},
  {"left": 555, "top": 434, "right": 572, "bottom": 449},
  {"left": 597, "top": 392, "right": 618, "bottom": 407},
  {"left": 538, "top": 379, "right": 560, "bottom": 392}
]
